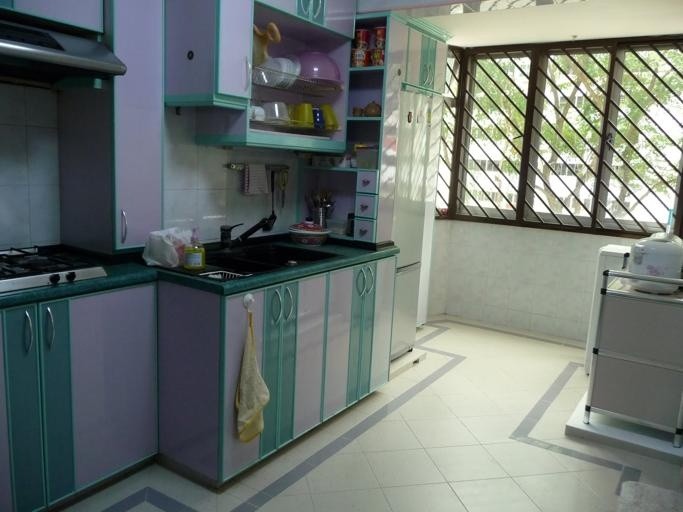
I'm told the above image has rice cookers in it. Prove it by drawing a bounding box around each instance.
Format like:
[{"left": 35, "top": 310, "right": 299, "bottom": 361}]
[{"left": 628, "top": 232, "right": 683, "bottom": 295}]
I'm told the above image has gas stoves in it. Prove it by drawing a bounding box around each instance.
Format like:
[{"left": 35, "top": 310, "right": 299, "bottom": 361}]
[{"left": 0, "top": 243, "right": 108, "bottom": 294}]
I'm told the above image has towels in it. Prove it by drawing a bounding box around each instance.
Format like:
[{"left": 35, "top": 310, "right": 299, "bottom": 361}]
[{"left": 233, "top": 307, "right": 272, "bottom": 442}]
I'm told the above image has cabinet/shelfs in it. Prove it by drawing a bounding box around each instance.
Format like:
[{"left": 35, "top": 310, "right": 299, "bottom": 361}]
[
  {"left": 163, "top": 0, "right": 255, "bottom": 112},
  {"left": 158, "top": 267, "right": 329, "bottom": 492},
  {"left": 402, "top": 26, "right": 455, "bottom": 97},
  {"left": 0, "top": 281, "right": 160, "bottom": 512},
  {"left": 322, "top": 254, "right": 399, "bottom": 430},
  {"left": 581, "top": 251, "right": 683, "bottom": 448},
  {"left": 296, "top": 169, "right": 396, "bottom": 244},
  {"left": 303, "top": 12, "right": 410, "bottom": 171},
  {"left": 193, "top": 1, "right": 357, "bottom": 155}
]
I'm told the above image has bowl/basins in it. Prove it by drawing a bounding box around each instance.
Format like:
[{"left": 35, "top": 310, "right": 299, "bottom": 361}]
[
  {"left": 288, "top": 218, "right": 333, "bottom": 247},
  {"left": 297, "top": 52, "right": 343, "bottom": 85}
]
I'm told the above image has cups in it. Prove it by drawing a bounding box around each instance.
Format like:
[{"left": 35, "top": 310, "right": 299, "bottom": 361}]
[{"left": 249, "top": 102, "right": 338, "bottom": 130}]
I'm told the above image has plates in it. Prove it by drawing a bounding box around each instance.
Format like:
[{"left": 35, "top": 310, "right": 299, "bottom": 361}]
[{"left": 254, "top": 55, "right": 301, "bottom": 89}]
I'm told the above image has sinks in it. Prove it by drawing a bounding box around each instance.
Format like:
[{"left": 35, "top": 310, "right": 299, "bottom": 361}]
[{"left": 252, "top": 245, "right": 339, "bottom": 265}]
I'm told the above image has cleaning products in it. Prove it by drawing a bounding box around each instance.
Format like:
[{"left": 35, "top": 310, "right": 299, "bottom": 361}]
[{"left": 183, "top": 228, "right": 206, "bottom": 269}]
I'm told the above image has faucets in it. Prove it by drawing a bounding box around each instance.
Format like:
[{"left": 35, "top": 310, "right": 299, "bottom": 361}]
[{"left": 220, "top": 218, "right": 271, "bottom": 247}]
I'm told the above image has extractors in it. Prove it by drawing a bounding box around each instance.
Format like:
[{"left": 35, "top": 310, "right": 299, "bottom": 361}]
[{"left": 0, "top": 16, "right": 126, "bottom": 93}]
